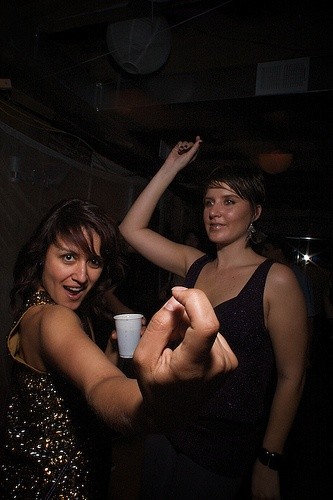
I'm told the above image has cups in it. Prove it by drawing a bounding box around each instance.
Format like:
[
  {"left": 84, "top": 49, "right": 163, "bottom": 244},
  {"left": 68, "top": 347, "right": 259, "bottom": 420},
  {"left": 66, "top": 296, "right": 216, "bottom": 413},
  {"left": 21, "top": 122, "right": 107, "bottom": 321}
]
[{"left": 114, "top": 314, "right": 143, "bottom": 359}]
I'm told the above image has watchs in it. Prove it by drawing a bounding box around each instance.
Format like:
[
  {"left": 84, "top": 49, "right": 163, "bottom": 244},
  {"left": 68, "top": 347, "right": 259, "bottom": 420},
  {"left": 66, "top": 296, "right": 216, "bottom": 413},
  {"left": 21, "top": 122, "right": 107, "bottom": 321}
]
[{"left": 258, "top": 447, "right": 284, "bottom": 471}]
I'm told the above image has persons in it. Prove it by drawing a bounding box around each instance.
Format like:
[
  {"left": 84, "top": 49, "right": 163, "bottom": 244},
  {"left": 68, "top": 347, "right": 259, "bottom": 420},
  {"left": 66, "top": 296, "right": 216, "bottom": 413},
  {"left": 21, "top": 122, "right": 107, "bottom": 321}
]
[
  {"left": 261, "top": 235, "right": 314, "bottom": 347},
  {"left": 101, "top": 222, "right": 141, "bottom": 314},
  {"left": 118, "top": 136, "right": 308, "bottom": 500},
  {"left": 2, "top": 200, "right": 237, "bottom": 498}
]
